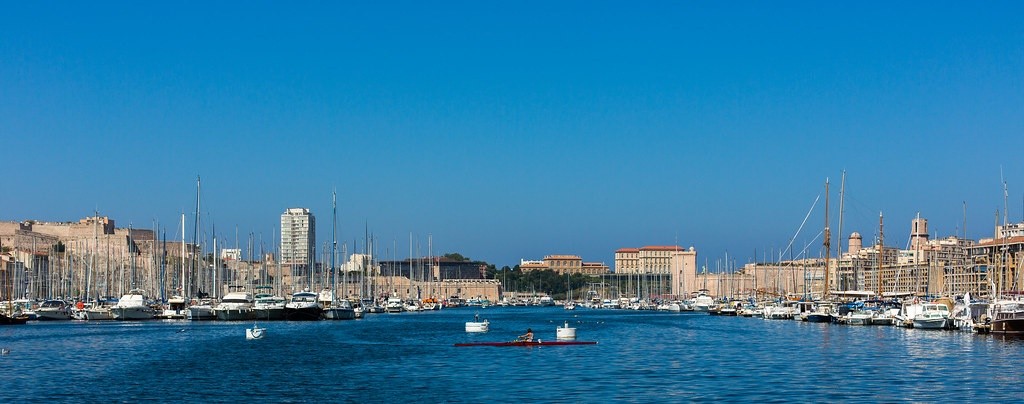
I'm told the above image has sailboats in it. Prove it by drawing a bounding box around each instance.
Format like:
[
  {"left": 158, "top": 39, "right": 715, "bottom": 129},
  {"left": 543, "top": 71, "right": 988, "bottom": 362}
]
[{"left": 2, "top": 173, "right": 1024, "bottom": 346}]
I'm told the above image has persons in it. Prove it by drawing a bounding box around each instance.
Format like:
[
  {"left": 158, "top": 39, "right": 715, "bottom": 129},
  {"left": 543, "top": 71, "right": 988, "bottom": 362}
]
[{"left": 518, "top": 328, "right": 534, "bottom": 342}]
